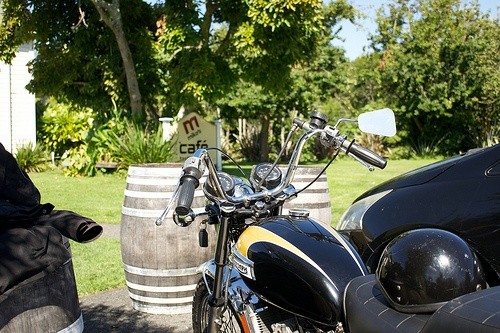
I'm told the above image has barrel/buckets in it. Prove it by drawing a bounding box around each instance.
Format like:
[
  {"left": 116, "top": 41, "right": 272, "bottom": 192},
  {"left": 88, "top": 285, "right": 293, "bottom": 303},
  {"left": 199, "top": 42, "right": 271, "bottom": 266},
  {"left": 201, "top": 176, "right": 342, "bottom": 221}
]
[
  {"left": 250, "top": 164, "right": 331, "bottom": 225},
  {"left": 119, "top": 163, "right": 215, "bottom": 314},
  {"left": 0, "top": 236, "right": 85, "bottom": 333}
]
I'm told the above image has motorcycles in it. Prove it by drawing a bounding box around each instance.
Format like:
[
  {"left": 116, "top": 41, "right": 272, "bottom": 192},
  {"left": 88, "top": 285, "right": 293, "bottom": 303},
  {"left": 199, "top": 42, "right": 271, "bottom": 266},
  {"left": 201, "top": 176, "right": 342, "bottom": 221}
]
[{"left": 155, "top": 106, "right": 500, "bottom": 333}]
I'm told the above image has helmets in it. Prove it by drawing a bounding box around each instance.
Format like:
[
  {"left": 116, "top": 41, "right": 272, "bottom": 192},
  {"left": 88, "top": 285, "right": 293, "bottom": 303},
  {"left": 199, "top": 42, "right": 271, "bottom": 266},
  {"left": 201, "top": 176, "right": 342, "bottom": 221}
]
[{"left": 376, "top": 229, "right": 491, "bottom": 313}]
